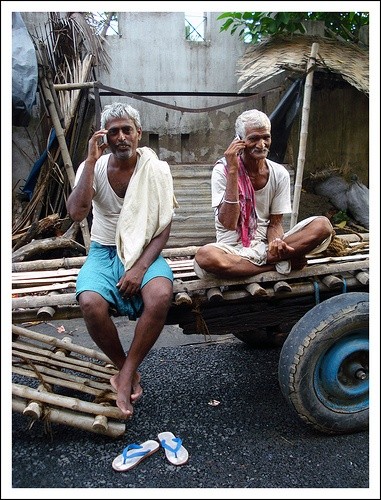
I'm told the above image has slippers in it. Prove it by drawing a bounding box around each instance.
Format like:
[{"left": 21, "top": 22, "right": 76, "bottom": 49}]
[
  {"left": 157, "top": 431, "right": 189, "bottom": 466},
  {"left": 111, "top": 439, "right": 160, "bottom": 472}
]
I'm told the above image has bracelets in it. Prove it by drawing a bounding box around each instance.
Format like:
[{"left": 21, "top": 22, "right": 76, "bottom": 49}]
[{"left": 223, "top": 198, "right": 240, "bottom": 205}]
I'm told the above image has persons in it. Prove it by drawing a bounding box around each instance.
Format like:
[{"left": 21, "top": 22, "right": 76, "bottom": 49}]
[
  {"left": 65, "top": 101, "right": 176, "bottom": 416},
  {"left": 194, "top": 109, "right": 335, "bottom": 280}
]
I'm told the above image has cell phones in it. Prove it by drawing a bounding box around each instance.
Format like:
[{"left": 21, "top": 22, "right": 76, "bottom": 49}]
[
  {"left": 236, "top": 134, "right": 243, "bottom": 154},
  {"left": 98, "top": 132, "right": 108, "bottom": 147}
]
